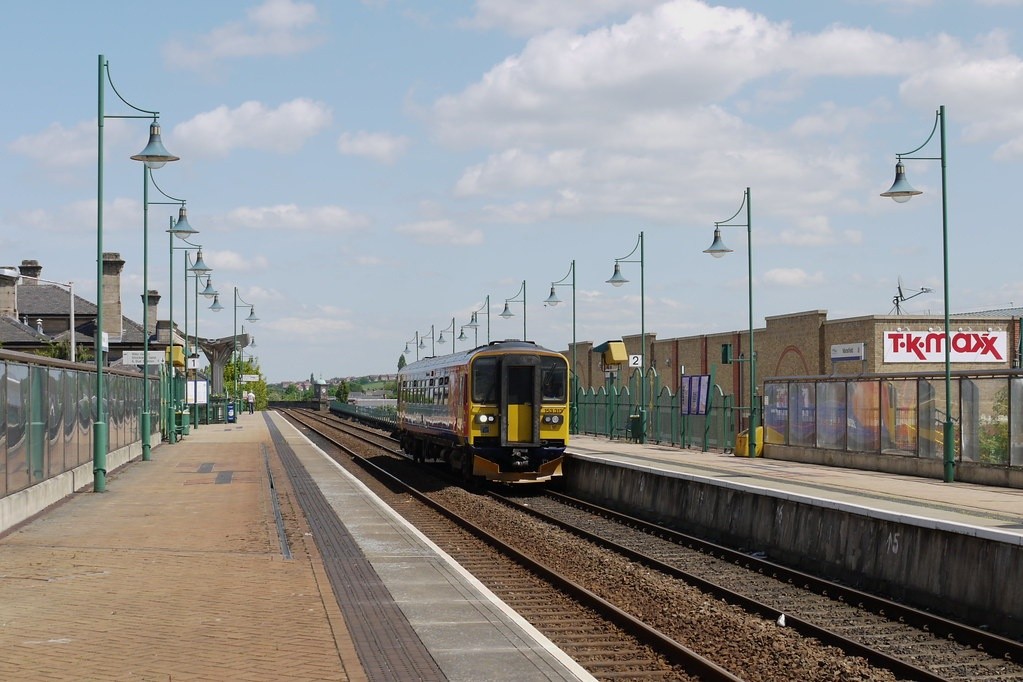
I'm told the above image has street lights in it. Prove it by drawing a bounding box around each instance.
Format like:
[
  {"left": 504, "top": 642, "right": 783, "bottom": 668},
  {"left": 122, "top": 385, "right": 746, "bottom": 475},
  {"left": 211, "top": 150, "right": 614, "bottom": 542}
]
[
  {"left": 419, "top": 324, "right": 435, "bottom": 357},
  {"left": 456, "top": 311, "right": 478, "bottom": 348},
  {"left": 544, "top": 259, "right": 577, "bottom": 436},
  {"left": 436, "top": 317, "right": 456, "bottom": 354},
  {"left": 91, "top": 54, "right": 178, "bottom": 493},
  {"left": 193, "top": 273, "right": 224, "bottom": 430},
  {"left": 885, "top": 104, "right": 961, "bottom": 485},
  {"left": 403, "top": 331, "right": 420, "bottom": 362},
  {"left": 704, "top": 186, "right": 761, "bottom": 458},
  {"left": 232, "top": 286, "right": 259, "bottom": 418},
  {"left": 606, "top": 230, "right": 649, "bottom": 444},
  {"left": 183, "top": 250, "right": 219, "bottom": 432},
  {"left": 167, "top": 216, "right": 212, "bottom": 444},
  {"left": 502, "top": 280, "right": 528, "bottom": 341},
  {"left": 467, "top": 294, "right": 491, "bottom": 344},
  {"left": 139, "top": 165, "right": 197, "bottom": 462}
]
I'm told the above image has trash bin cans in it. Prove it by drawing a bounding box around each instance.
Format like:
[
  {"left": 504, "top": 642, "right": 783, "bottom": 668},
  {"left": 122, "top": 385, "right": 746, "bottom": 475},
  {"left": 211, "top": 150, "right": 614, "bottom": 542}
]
[
  {"left": 735, "top": 426, "right": 764, "bottom": 458},
  {"left": 175, "top": 411, "right": 190, "bottom": 435}
]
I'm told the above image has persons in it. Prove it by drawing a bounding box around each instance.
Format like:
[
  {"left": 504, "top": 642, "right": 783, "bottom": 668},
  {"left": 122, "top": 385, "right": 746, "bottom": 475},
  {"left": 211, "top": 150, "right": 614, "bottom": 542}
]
[{"left": 247, "top": 390, "right": 256, "bottom": 414}]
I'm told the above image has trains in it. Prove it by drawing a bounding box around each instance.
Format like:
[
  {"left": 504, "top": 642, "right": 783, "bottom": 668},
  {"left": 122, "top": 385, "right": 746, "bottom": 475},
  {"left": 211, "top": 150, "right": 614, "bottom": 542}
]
[{"left": 394, "top": 340, "right": 570, "bottom": 489}]
